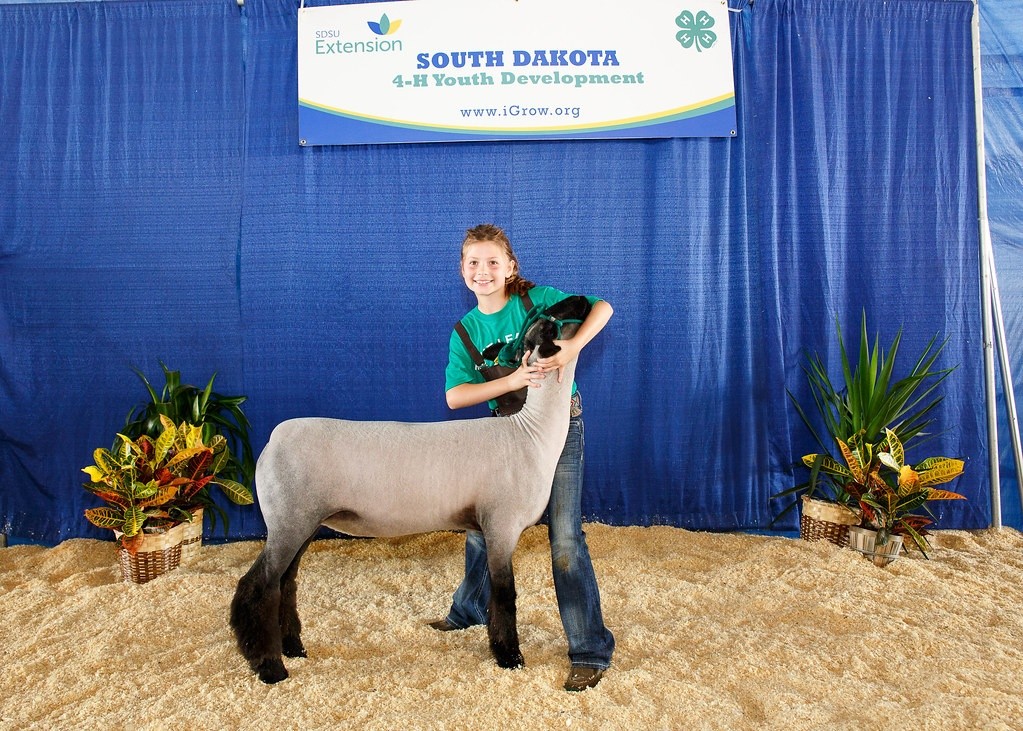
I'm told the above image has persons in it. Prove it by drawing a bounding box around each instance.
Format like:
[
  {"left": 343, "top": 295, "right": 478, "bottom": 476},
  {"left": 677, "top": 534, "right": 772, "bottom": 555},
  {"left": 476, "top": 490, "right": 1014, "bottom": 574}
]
[{"left": 429, "top": 223, "right": 613, "bottom": 692}]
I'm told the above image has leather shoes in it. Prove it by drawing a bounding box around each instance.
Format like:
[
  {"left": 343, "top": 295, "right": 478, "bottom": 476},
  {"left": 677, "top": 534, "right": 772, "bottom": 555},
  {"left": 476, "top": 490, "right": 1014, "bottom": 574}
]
[
  {"left": 428, "top": 620, "right": 453, "bottom": 632},
  {"left": 562, "top": 666, "right": 603, "bottom": 691}
]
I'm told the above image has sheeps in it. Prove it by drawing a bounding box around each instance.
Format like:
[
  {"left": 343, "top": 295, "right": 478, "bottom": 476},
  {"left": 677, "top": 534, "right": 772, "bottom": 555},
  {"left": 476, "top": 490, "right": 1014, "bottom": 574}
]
[{"left": 229, "top": 294, "right": 592, "bottom": 684}]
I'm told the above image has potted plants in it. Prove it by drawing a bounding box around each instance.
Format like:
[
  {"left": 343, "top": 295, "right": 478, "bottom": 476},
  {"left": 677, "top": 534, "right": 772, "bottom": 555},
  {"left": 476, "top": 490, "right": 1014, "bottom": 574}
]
[
  {"left": 768, "top": 306, "right": 969, "bottom": 572},
  {"left": 77, "top": 357, "right": 256, "bottom": 585}
]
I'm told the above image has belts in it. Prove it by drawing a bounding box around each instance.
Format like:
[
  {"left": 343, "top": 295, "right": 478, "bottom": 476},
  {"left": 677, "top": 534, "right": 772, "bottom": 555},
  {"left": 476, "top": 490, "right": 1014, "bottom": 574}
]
[{"left": 571, "top": 393, "right": 580, "bottom": 407}]
